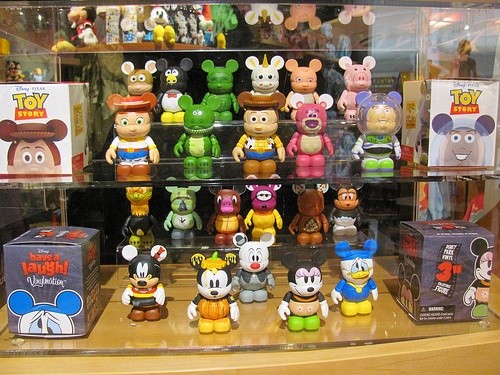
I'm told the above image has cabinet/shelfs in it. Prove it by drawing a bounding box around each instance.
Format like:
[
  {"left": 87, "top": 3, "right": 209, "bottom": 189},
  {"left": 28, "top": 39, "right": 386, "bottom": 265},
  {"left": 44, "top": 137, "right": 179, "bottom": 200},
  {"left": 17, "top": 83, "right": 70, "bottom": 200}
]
[{"left": 0, "top": 43, "right": 500, "bottom": 194}]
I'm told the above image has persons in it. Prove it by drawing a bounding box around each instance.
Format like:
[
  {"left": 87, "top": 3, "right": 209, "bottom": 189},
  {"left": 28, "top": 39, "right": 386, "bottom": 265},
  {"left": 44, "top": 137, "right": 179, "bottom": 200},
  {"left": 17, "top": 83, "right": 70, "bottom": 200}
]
[
  {"left": 351, "top": 91, "right": 403, "bottom": 168},
  {"left": 457, "top": 40, "right": 477, "bottom": 77},
  {"left": 29, "top": 67, "right": 47, "bottom": 82},
  {"left": 105, "top": 93, "right": 160, "bottom": 174},
  {"left": 232, "top": 91, "right": 287, "bottom": 172}
]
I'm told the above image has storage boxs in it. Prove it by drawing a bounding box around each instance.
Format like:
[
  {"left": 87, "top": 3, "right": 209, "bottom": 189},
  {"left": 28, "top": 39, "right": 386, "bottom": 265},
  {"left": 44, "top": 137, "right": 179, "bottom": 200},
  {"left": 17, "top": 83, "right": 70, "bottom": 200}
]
[
  {"left": 3, "top": 226, "right": 102, "bottom": 340},
  {"left": 400, "top": 77, "right": 500, "bottom": 175},
  {"left": 0, "top": 81, "right": 92, "bottom": 182},
  {"left": 398, "top": 220, "right": 495, "bottom": 327}
]
